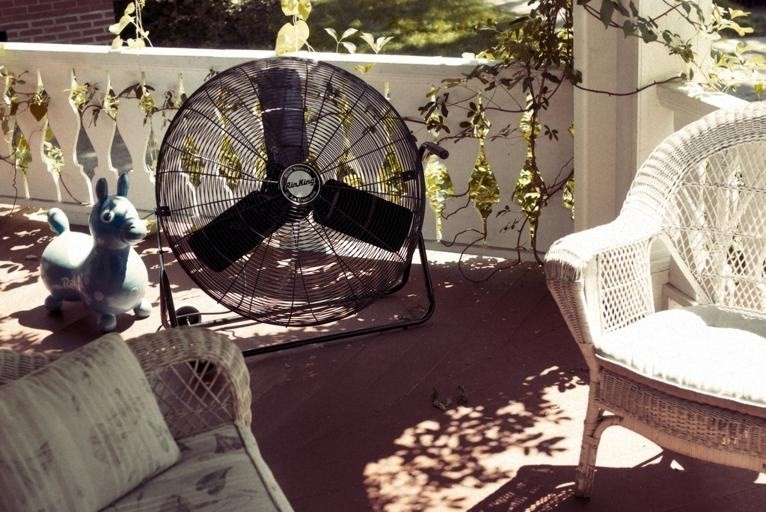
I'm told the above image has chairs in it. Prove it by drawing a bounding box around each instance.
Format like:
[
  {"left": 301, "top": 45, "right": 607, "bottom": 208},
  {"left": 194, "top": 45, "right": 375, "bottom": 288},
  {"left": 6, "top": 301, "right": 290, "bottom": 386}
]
[
  {"left": 0, "top": 324, "right": 296, "bottom": 511},
  {"left": 542, "top": 98, "right": 766, "bottom": 505}
]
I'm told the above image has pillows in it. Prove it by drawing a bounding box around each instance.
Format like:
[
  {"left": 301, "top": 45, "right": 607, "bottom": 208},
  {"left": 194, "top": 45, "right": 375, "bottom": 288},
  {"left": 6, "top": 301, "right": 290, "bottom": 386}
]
[{"left": 0, "top": 331, "right": 182, "bottom": 511}]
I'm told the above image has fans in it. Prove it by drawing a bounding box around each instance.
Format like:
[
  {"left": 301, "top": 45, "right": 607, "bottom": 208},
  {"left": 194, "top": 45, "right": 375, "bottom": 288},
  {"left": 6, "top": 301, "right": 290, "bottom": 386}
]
[{"left": 154, "top": 55, "right": 438, "bottom": 374}]
